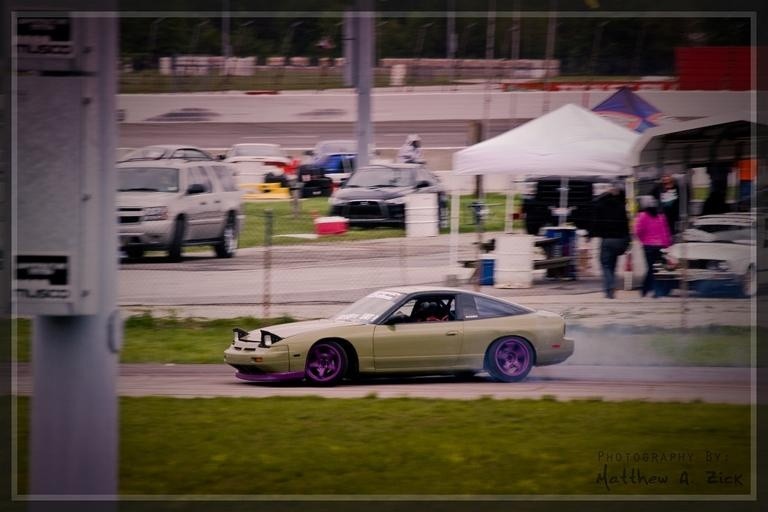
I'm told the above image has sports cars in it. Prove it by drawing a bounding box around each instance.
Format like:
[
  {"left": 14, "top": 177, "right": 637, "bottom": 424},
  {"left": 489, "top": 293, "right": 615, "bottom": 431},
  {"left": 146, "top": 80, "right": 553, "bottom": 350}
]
[{"left": 223, "top": 286, "right": 574, "bottom": 387}]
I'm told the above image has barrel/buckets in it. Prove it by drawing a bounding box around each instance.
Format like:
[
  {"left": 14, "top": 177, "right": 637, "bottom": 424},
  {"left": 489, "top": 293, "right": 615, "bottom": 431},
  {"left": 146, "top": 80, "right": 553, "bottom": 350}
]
[
  {"left": 478, "top": 254, "right": 496, "bottom": 284},
  {"left": 405, "top": 193, "right": 439, "bottom": 237},
  {"left": 543, "top": 225, "right": 577, "bottom": 284}
]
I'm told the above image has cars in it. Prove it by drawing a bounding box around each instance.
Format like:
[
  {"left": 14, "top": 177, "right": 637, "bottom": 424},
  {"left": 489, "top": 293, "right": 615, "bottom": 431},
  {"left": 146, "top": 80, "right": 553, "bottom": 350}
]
[{"left": 652, "top": 215, "right": 768, "bottom": 297}]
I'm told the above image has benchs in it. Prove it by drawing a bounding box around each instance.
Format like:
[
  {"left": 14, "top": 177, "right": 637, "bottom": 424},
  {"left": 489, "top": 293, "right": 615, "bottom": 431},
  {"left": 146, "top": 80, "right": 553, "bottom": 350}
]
[{"left": 462, "top": 235, "right": 575, "bottom": 285}]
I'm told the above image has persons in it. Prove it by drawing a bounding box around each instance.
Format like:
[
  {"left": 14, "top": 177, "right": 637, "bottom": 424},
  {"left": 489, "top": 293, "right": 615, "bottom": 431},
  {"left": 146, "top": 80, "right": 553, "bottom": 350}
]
[
  {"left": 396, "top": 135, "right": 425, "bottom": 163},
  {"left": 589, "top": 186, "right": 629, "bottom": 299},
  {"left": 633, "top": 195, "right": 674, "bottom": 298},
  {"left": 424, "top": 305, "right": 449, "bottom": 321},
  {"left": 649, "top": 175, "right": 680, "bottom": 228}
]
